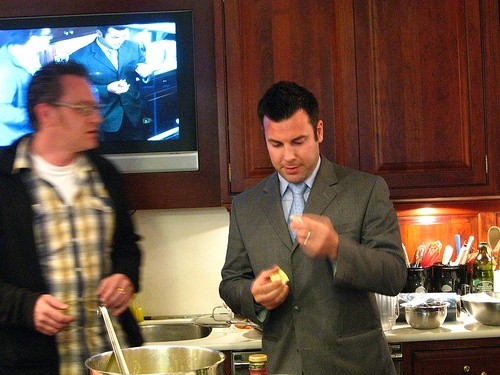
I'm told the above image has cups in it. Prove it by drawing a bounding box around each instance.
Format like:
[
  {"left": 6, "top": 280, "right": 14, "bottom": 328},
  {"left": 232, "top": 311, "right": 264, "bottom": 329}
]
[
  {"left": 374, "top": 291, "right": 400, "bottom": 335},
  {"left": 431, "top": 262, "right": 468, "bottom": 294},
  {"left": 407, "top": 266, "right": 433, "bottom": 293}
]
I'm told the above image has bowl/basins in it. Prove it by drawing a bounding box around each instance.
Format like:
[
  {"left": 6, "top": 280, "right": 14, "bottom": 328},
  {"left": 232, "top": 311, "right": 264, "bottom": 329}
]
[
  {"left": 397, "top": 291, "right": 459, "bottom": 322},
  {"left": 400, "top": 302, "right": 450, "bottom": 330},
  {"left": 461, "top": 292, "right": 500, "bottom": 326}
]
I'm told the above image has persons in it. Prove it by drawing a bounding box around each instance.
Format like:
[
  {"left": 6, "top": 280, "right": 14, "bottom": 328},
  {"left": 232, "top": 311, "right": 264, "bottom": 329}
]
[
  {"left": 0, "top": 28, "right": 54, "bottom": 147},
  {"left": 68, "top": 25, "right": 149, "bottom": 141},
  {"left": 219, "top": 80, "right": 407, "bottom": 375},
  {"left": 0, "top": 60, "right": 144, "bottom": 375}
]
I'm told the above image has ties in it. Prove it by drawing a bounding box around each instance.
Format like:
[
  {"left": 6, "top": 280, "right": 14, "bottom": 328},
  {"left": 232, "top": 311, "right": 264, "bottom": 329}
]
[
  {"left": 108, "top": 49, "right": 117, "bottom": 69},
  {"left": 286, "top": 182, "right": 308, "bottom": 244}
]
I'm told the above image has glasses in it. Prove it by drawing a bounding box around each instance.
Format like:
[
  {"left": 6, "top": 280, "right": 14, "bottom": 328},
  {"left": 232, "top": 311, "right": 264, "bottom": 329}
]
[
  {"left": 33, "top": 99, "right": 100, "bottom": 116},
  {"left": 28, "top": 32, "right": 53, "bottom": 40}
]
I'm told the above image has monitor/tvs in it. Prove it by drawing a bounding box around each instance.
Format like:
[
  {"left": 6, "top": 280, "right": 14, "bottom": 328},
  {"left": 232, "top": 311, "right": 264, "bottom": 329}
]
[{"left": 0, "top": 8, "right": 199, "bottom": 173}]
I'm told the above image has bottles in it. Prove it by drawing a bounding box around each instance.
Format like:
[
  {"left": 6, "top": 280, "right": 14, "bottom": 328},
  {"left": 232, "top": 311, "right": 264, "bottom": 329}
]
[
  {"left": 249, "top": 354, "right": 268, "bottom": 375},
  {"left": 472, "top": 241, "right": 494, "bottom": 293},
  {"left": 462, "top": 284, "right": 471, "bottom": 296}
]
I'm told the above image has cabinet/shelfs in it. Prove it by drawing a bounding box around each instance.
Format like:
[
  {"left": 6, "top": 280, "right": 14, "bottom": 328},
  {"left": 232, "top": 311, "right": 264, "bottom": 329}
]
[
  {"left": 212, "top": 0, "right": 500, "bottom": 215},
  {"left": 401, "top": 336, "right": 500, "bottom": 375}
]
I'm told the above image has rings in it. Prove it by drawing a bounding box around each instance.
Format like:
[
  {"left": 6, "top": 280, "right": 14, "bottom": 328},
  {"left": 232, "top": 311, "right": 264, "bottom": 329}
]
[
  {"left": 304, "top": 238, "right": 308, "bottom": 246},
  {"left": 307, "top": 231, "right": 312, "bottom": 240},
  {"left": 117, "top": 288, "right": 127, "bottom": 294}
]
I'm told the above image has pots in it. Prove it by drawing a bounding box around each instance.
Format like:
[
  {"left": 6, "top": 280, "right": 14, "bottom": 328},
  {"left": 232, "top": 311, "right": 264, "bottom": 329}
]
[{"left": 84, "top": 344, "right": 222, "bottom": 375}]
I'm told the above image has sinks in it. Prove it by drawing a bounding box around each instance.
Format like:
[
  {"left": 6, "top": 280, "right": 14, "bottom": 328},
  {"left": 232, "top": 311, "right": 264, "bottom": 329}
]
[{"left": 138, "top": 322, "right": 212, "bottom": 341}]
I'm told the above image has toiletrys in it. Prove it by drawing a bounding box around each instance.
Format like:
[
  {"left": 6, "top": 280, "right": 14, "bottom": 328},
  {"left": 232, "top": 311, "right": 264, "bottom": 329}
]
[{"left": 132, "top": 294, "right": 144, "bottom": 322}]
